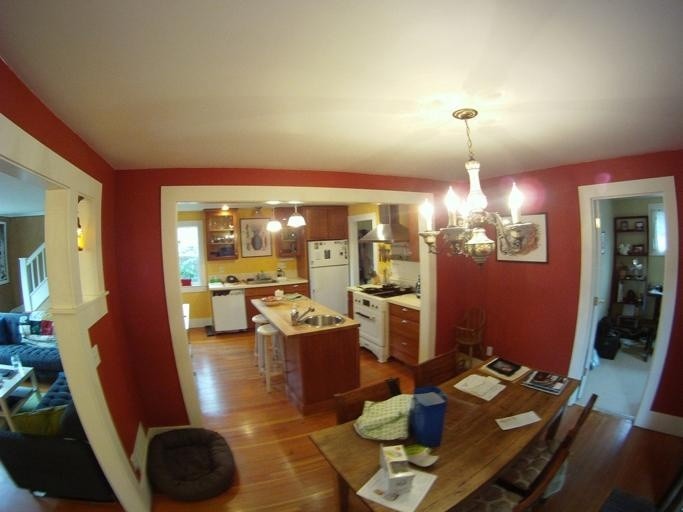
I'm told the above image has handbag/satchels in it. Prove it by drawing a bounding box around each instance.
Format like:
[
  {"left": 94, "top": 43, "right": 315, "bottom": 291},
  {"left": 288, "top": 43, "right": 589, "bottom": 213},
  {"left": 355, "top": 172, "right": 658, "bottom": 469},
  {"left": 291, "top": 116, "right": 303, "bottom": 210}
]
[{"left": 409, "top": 386, "right": 447, "bottom": 448}]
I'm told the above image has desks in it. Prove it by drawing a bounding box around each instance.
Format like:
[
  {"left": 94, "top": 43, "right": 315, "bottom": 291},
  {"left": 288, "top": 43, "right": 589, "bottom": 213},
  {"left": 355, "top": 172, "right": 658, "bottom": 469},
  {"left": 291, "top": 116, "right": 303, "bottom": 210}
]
[
  {"left": 310, "top": 355, "right": 582, "bottom": 512},
  {"left": 641, "top": 287, "right": 663, "bottom": 362},
  {"left": 0, "top": 364, "right": 43, "bottom": 431}
]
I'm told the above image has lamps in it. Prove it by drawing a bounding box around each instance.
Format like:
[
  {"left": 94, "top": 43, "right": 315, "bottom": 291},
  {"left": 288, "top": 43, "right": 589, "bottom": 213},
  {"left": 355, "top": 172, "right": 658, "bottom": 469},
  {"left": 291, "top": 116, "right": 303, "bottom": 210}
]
[
  {"left": 287, "top": 205, "right": 306, "bottom": 229},
  {"left": 266, "top": 207, "right": 282, "bottom": 232},
  {"left": 417, "top": 108, "right": 538, "bottom": 267}
]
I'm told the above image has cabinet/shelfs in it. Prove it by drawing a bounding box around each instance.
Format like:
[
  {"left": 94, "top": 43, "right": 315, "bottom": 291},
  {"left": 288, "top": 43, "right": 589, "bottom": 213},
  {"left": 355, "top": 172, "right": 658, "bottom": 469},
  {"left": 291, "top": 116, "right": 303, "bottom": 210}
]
[
  {"left": 204, "top": 211, "right": 240, "bottom": 261},
  {"left": 612, "top": 215, "right": 649, "bottom": 326},
  {"left": 387, "top": 302, "right": 420, "bottom": 367},
  {"left": 272, "top": 207, "right": 304, "bottom": 257},
  {"left": 299, "top": 206, "right": 349, "bottom": 242},
  {"left": 244, "top": 283, "right": 311, "bottom": 331}
]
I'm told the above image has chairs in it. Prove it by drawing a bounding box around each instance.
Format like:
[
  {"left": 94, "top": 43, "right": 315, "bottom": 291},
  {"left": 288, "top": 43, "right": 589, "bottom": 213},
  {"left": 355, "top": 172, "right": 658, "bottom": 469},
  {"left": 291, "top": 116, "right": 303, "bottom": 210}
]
[
  {"left": 413, "top": 349, "right": 463, "bottom": 390},
  {"left": 452, "top": 304, "right": 488, "bottom": 358},
  {"left": 444, "top": 447, "right": 571, "bottom": 512},
  {"left": 333, "top": 376, "right": 402, "bottom": 511},
  {"left": 497, "top": 393, "right": 597, "bottom": 502}
]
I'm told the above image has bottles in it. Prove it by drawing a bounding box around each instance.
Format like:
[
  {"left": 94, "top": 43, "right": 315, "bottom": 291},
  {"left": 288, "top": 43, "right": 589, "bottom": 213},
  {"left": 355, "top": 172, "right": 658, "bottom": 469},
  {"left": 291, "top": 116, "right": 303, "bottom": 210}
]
[{"left": 415, "top": 273, "right": 420, "bottom": 298}]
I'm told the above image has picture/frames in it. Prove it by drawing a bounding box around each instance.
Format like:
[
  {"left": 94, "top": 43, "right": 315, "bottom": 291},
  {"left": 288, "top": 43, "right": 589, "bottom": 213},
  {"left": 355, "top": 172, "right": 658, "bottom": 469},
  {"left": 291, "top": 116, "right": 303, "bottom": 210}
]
[
  {"left": 239, "top": 218, "right": 273, "bottom": 258},
  {"left": 495, "top": 213, "right": 548, "bottom": 263}
]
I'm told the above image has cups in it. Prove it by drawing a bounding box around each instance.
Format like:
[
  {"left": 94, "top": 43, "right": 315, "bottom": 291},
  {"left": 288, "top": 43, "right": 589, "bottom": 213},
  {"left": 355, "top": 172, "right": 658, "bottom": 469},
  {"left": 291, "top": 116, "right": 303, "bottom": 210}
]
[{"left": 10, "top": 354, "right": 23, "bottom": 374}]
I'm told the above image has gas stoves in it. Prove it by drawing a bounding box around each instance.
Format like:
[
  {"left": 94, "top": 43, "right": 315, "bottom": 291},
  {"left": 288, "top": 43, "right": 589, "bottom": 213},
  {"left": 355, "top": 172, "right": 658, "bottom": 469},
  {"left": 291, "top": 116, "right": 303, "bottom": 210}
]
[
  {"left": 358, "top": 203, "right": 411, "bottom": 243},
  {"left": 353, "top": 279, "right": 416, "bottom": 309}
]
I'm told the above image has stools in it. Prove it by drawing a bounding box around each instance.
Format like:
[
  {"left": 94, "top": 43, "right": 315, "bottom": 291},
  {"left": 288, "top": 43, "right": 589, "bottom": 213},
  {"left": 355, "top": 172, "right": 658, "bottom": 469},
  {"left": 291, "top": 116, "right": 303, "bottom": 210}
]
[
  {"left": 250, "top": 314, "right": 269, "bottom": 359},
  {"left": 256, "top": 326, "right": 279, "bottom": 393}
]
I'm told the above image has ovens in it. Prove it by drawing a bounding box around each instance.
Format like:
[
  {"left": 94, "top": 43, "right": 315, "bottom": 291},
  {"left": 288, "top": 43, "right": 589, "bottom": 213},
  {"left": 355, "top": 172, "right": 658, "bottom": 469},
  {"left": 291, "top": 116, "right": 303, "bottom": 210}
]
[{"left": 353, "top": 305, "right": 390, "bottom": 347}]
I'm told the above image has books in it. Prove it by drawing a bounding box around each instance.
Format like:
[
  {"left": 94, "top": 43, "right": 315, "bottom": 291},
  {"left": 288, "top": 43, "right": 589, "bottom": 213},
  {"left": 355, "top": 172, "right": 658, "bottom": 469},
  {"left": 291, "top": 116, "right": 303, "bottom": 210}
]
[{"left": 483, "top": 357, "right": 570, "bottom": 396}]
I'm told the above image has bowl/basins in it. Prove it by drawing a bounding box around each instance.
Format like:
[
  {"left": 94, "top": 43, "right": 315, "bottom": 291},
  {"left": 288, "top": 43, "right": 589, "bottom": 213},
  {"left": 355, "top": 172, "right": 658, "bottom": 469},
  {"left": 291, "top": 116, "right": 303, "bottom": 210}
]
[
  {"left": 260, "top": 295, "right": 283, "bottom": 307},
  {"left": 355, "top": 285, "right": 379, "bottom": 293}
]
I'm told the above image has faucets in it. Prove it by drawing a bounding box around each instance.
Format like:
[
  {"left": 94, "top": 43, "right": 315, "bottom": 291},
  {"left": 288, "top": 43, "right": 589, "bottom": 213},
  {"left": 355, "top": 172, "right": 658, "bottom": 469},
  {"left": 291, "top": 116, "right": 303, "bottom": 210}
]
[{"left": 290, "top": 307, "right": 315, "bottom": 326}]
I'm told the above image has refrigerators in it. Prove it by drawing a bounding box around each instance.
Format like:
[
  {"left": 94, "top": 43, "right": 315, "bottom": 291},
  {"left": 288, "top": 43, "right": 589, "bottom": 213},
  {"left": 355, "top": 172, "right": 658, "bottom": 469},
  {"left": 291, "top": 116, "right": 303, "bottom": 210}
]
[{"left": 305, "top": 239, "right": 351, "bottom": 319}]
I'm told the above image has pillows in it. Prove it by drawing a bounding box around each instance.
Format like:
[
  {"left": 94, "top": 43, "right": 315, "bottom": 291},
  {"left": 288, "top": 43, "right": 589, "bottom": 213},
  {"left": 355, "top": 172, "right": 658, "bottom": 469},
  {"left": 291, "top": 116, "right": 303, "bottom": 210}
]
[{"left": 9, "top": 406, "right": 66, "bottom": 434}]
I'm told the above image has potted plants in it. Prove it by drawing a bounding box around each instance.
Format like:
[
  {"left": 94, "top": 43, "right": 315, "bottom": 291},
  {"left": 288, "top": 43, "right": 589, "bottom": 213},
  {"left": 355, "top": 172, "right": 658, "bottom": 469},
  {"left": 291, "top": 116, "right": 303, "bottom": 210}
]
[{"left": 180, "top": 270, "right": 193, "bottom": 286}]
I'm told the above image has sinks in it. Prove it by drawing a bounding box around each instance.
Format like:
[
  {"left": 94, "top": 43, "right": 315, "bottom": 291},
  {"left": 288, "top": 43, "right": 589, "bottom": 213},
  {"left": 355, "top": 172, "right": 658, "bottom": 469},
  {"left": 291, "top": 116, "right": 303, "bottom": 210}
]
[
  {"left": 301, "top": 314, "right": 345, "bottom": 328},
  {"left": 242, "top": 276, "right": 278, "bottom": 286}
]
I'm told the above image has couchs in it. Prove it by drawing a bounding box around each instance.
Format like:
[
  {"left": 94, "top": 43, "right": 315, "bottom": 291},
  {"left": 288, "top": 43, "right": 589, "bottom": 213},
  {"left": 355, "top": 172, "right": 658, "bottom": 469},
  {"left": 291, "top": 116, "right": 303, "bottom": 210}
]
[
  {"left": 0, "top": 371, "right": 119, "bottom": 504},
  {"left": 0, "top": 310, "right": 62, "bottom": 382}
]
